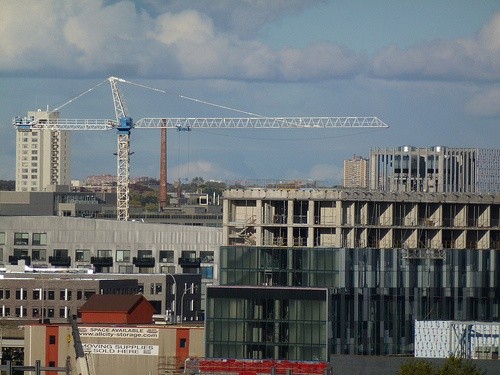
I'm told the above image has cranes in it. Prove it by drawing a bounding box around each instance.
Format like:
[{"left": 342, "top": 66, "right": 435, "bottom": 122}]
[{"left": 14, "top": 74, "right": 390, "bottom": 222}]
